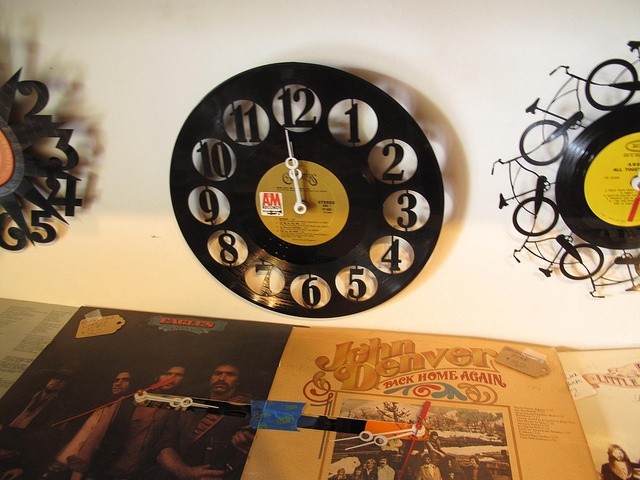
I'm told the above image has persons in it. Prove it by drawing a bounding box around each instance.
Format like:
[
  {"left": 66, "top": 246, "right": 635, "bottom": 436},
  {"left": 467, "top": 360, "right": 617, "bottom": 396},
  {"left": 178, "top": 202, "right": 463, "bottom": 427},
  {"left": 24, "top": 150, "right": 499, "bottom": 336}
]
[
  {"left": 1, "top": 368, "right": 138, "bottom": 479},
  {"left": 0, "top": 370, "right": 75, "bottom": 480},
  {"left": 334, "top": 430, "right": 493, "bottom": 480},
  {"left": 601, "top": 444, "right": 640, "bottom": 480},
  {"left": 149, "top": 359, "right": 257, "bottom": 479},
  {"left": 85, "top": 356, "right": 193, "bottom": 479}
]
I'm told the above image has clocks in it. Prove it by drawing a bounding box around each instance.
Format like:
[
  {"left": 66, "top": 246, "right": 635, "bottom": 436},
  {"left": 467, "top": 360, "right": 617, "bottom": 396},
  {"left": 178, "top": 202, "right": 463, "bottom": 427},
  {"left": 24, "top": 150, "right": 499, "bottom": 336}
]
[
  {"left": 490, "top": 34, "right": 639, "bottom": 299},
  {"left": 0, "top": 65, "right": 85, "bottom": 253},
  {"left": 167, "top": 62, "right": 445, "bottom": 317}
]
